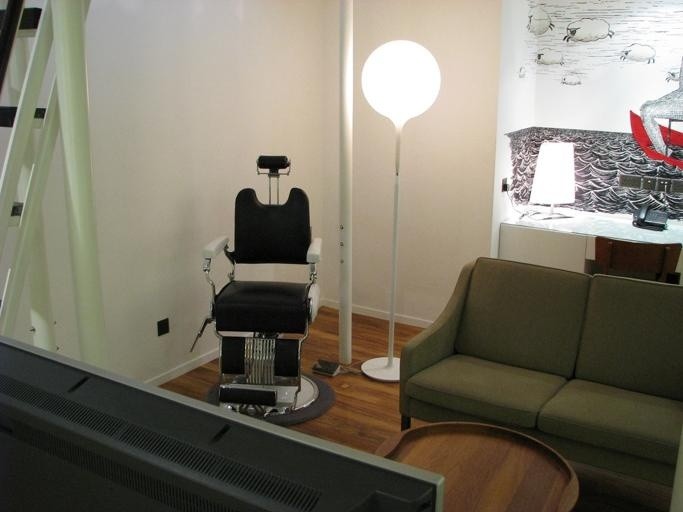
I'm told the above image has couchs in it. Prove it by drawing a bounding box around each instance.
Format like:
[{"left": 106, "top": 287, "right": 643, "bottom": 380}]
[{"left": 399, "top": 257, "right": 682, "bottom": 512}]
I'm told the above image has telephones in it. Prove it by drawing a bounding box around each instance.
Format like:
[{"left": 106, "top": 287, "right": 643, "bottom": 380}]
[{"left": 637, "top": 204, "right": 668, "bottom": 226}]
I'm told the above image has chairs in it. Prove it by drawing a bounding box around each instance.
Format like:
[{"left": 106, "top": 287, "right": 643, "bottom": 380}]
[
  {"left": 594, "top": 236, "right": 682, "bottom": 284},
  {"left": 203, "top": 155, "right": 336, "bottom": 428}
]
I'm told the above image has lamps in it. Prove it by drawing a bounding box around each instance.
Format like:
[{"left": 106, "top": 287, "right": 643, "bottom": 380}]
[
  {"left": 360, "top": 40, "right": 444, "bottom": 384},
  {"left": 528, "top": 140, "right": 577, "bottom": 221}
]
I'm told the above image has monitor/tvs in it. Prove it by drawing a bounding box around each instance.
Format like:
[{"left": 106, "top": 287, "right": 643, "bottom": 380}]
[{"left": 0, "top": 335, "right": 444, "bottom": 512}]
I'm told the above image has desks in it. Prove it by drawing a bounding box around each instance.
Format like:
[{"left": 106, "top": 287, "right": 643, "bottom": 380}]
[{"left": 500, "top": 205, "right": 683, "bottom": 273}]
